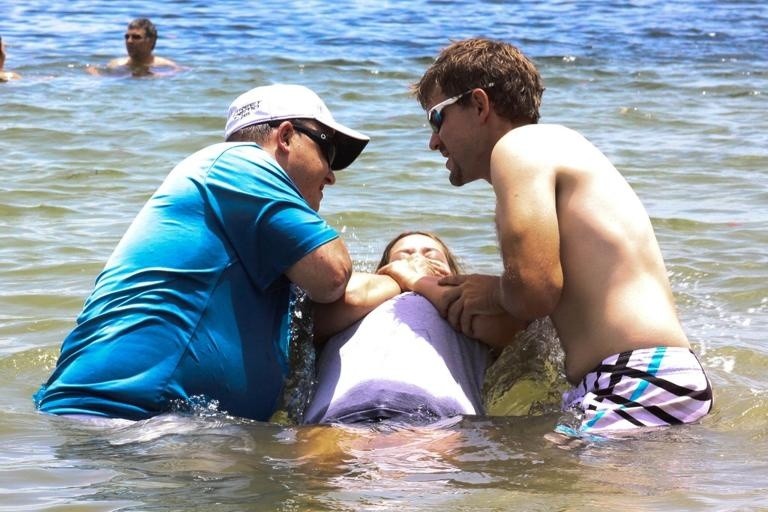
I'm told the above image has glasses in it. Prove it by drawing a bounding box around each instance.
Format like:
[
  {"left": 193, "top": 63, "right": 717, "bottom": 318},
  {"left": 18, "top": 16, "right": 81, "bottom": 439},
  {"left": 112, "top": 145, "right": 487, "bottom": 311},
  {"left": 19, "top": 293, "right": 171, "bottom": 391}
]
[
  {"left": 427, "top": 82, "right": 495, "bottom": 133},
  {"left": 271, "top": 119, "right": 336, "bottom": 168}
]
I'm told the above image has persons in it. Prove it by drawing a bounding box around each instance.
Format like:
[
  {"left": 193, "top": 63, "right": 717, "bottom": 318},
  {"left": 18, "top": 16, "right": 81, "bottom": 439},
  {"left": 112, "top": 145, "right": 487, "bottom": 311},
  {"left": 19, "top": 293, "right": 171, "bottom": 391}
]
[
  {"left": 0, "top": 36, "right": 22, "bottom": 82},
  {"left": 108, "top": 19, "right": 177, "bottom": 68}
]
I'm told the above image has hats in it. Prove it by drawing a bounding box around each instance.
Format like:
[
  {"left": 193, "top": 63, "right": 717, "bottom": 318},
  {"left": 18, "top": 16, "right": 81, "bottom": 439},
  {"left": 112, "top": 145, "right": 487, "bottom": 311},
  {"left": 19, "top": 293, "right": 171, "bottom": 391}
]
[{"left": 225, "top": 84, "right": 370, "bottom": 170}]
